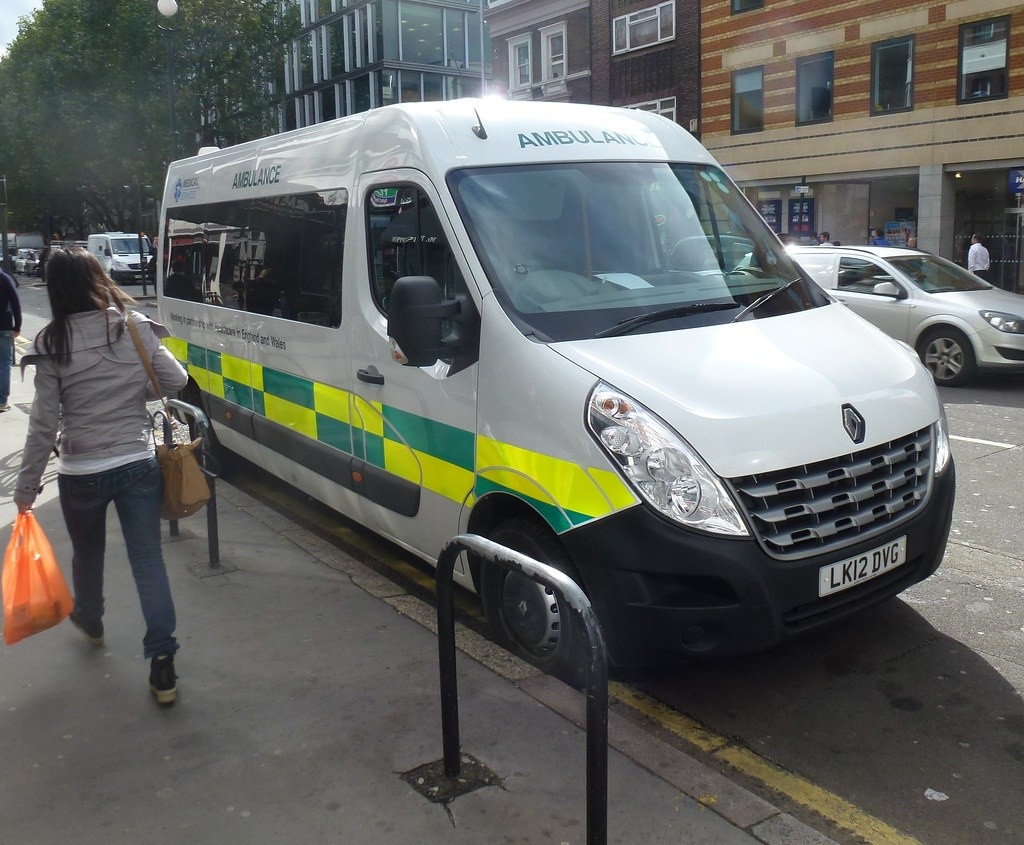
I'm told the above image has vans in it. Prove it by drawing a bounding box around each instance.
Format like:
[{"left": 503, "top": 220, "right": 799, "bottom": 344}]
[{"left": 89, "top": 232, "right": 152, "bottom": 285}]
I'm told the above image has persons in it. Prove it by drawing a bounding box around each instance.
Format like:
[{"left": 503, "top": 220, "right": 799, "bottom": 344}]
[
  {"left": 163, "top": 260, "right": 198, "bottom": 302},
  {"left": 908, "top": 237, "right": 922, "bottom": 272},
  {"left": 0, "top": 236, "right": 189, "bottom": 702},
  {"left": 968, "top": 232, "right": 989, "bottom": 283},
  {"left": 872, "top": 229, "right": 893, "bottom": 248},
  {"left": 809, "top": 232, "right": 841, "bottom": 245}
]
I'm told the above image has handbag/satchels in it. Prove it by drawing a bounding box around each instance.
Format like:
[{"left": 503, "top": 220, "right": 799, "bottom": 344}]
[
  {"left": 154, "top": 443, "right": 211, "bottom": 519},
  {"left": 1, "top": 509, "right": 74, "bottom": 646}
]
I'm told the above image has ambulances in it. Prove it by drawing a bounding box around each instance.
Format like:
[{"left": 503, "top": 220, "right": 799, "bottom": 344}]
[{"left": 155, "top": 94, "right": 959, "bottom": 684}]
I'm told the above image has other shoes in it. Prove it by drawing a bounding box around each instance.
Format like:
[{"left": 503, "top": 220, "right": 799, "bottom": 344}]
[
  {"left": 68, "top": 595, "right": 104, "bottom": 644},
  {"left": 149, "top": 655, "right": 178, "bottom": 704},
  {"left": 0, "top": 401, "right": 10, "bottom": 412}
]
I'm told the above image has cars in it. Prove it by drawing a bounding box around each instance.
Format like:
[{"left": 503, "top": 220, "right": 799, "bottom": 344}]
[
  {"left": 731, "top": 246, "right": 1024, "bottom": 385},
  {"left": 15, "top": 248, "right": 48, "bottom": 278},
  {"left": 667, "top": 233, "right": 757, "bottom": 270}
]
